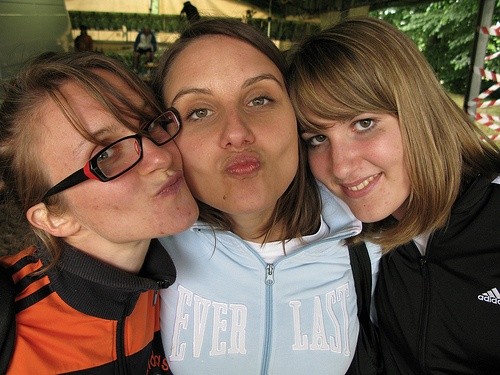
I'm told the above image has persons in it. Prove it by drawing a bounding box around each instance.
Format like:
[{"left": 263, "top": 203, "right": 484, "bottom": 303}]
[
  {"left": 73, "top": 25, "right": 94, "bottom": 54},
  {"left": 149, "top": 17, "right": 385, "bottom": 375},
  {"left": 132, "top": 24, "right": 157, "bottom": 77},
  {"left": 0, "top": 52, "right": 202, "bottom": 375},
  {"left": 286, "top": 17, "right": 500, "bottom": 374}
]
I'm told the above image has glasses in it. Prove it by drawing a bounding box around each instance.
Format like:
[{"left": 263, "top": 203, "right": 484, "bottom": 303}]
[{"left": 42, "top": 106, "right": 182, "bottom": 203}]
